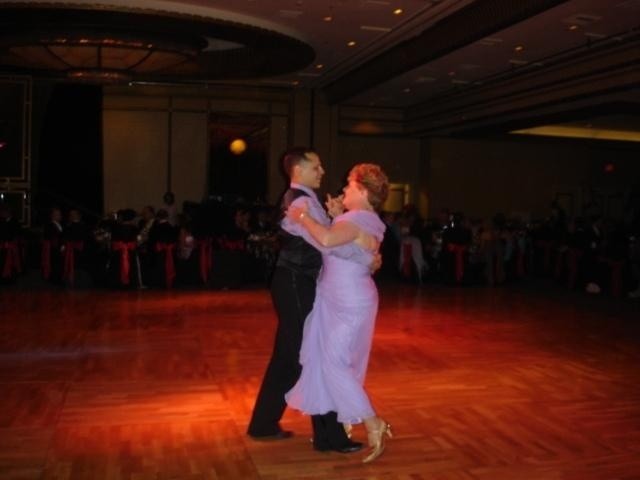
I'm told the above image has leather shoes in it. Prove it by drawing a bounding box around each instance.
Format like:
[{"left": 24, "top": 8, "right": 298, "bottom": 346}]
[
  {"left": 252, "top": 429, "right": 292, "bottom": 439},
  {"left": 315, "top": 438, "right": 362, "bottom": 453}
]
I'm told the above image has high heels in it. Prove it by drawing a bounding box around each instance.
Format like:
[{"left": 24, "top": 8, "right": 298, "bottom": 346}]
[
  {"left": 363, "top": 420, "right": 393, "bottom": 464},
  {"left": 311, "top": 425, "right": 354, "bottom": 440}
]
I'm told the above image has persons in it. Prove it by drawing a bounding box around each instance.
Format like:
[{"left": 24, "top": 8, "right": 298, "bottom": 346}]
[
  {"left": 1, "top": 192, "right": 639, "bottom": 303},
  {"left": 283, "top": 161, "right": 393, "bottom": 465},
  {"left": 244, "top": 146, "right": 366, "bottom": 454}
]
